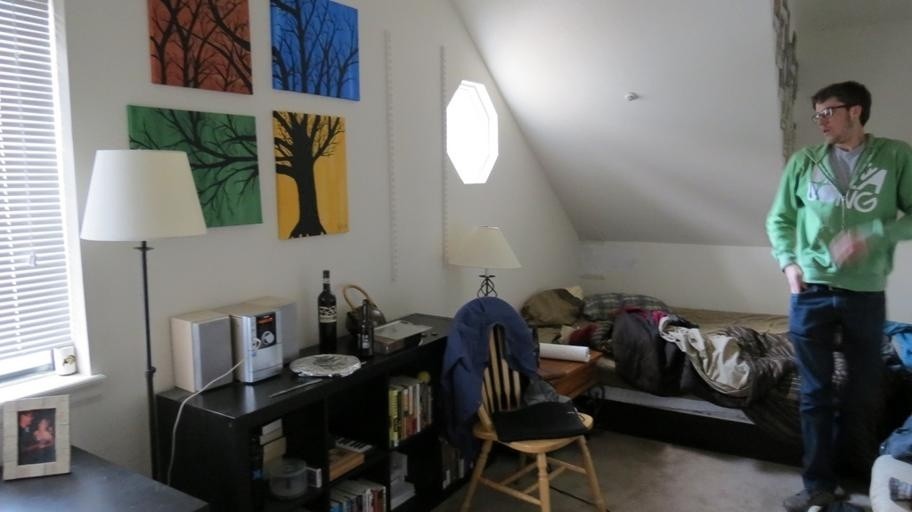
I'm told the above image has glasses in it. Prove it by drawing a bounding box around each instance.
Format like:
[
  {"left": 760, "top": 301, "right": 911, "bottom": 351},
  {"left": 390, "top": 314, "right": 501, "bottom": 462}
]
[{"left": 811, "top": 104, "right": 858, "bottom": 122}]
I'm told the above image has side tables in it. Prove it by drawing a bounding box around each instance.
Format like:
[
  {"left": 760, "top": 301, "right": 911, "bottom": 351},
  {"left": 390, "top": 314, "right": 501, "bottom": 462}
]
[{"left": 538, "top": 350, "right": 609, "bottom": 440}]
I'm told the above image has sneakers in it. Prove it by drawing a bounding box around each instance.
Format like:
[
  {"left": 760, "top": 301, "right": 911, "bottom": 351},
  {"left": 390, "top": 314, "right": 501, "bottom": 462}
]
[{"left": 784, "top": 489, "right": 834, "bottom": 512}]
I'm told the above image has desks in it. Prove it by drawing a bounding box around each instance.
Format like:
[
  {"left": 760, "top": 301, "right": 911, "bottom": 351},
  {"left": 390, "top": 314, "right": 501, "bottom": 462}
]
[{"left": 0, "top": 444, "right": 208, "bottom": 512}]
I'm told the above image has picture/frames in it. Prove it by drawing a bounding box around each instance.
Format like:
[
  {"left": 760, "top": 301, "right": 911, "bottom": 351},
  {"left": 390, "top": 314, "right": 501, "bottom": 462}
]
[{"left": 0, "top": 392, "right": 71, "bottom": 482}]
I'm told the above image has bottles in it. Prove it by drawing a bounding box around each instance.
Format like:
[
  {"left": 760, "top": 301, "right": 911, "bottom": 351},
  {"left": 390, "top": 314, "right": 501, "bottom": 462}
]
[
  {"left": 357, "top": 298, "right": 374, "bottom": 360},
  {"left": 317, "top": 270, "right": 337, "bottom": 353}
]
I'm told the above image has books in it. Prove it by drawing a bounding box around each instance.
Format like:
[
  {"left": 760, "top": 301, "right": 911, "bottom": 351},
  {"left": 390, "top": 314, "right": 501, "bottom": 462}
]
[
  {"left": 387, "top": 372, "right": 434, "bottom": 511},
  {"left": 327, "top": 445, "right": 388, "bottom": 512},
  {"left": 438, "top": 442, "right": 473, "bottom": 492},
  {"left": 369, "top": 319, "right": 432, "bottom": 345},
  {"left": 250, "top": 417, "right": 290, "bottom": 482}
]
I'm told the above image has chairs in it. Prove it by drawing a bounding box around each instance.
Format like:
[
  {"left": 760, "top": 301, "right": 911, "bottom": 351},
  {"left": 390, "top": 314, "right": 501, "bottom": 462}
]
[{"left": 446, "top": 296, "right": 608, "bottom": 512}]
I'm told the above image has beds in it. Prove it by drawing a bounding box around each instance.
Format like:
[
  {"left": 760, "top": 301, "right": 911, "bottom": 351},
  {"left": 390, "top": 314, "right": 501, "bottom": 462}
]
[{"left": 521, "top": 294, "right": 847, "bottom": 467}]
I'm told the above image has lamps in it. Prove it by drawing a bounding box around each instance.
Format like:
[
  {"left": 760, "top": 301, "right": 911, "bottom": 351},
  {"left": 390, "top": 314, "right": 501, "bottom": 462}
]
[
  {"left": 448, "top": 225, "right": 522, "bottom": 298},
  {"left": 79, "top": 149, "right": 208, "bottom": 481}
]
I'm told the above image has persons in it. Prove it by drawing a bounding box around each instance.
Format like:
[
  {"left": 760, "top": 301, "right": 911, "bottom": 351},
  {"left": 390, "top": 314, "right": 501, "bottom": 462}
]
[
  {"left": 761, "top": 78, "right": 912, "bottom": 511},
  {"left": 32, "top": 419, "right": 56, "bottom": 457},
  {"left": 16, "top": 411, "right": 42, "bottom": 466}
]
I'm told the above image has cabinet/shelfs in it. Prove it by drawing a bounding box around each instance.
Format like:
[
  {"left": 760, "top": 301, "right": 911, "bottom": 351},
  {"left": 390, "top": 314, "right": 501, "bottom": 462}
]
[{"left": 154, "top": 312, "right": 508, "bottom": 512}]
